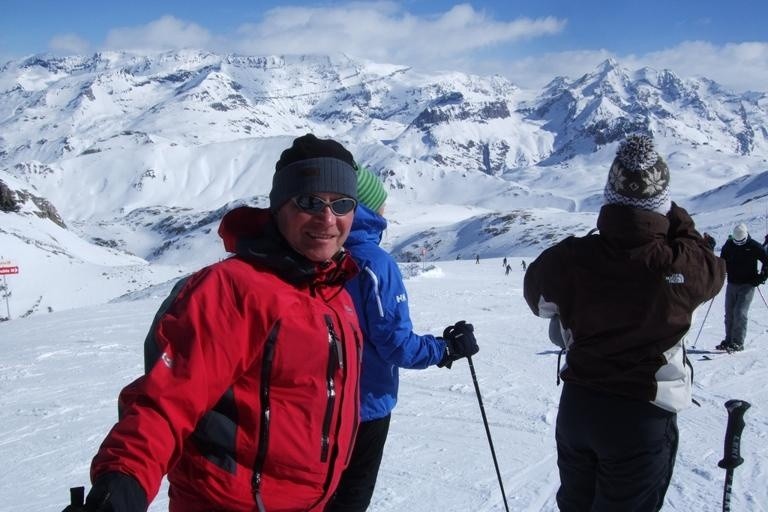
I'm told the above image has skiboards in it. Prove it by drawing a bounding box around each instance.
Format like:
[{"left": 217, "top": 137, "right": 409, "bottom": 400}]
[{"left": 689, "top": 349, "right": 731, "bottom": 358}]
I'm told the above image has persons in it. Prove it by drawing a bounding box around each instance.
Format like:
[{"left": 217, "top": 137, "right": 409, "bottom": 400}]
[
  {"left": 521, "top": 135, "right": 729, "bottom": 510},
  {"left": 66, "top": 131, "right": 366, "bottom": 508},
  {"left": 719, "top": 220, "right": 766, "bottom": 357},
  {"left": 320, "top": 158, "right": 480, "bottom": 508}
]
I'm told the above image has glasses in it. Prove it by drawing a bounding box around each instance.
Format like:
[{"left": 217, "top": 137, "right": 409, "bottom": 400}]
[{"left": 290, "top": 193, "right": 356, "bottom": 216}]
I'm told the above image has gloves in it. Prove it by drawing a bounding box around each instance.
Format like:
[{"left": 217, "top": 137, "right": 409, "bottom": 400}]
[
  {"left": 61, "top": 472, "right": 149, "bottom": 512},
  {"left": 749, "top": 271, "right": 767, "bottom": 286},
  {"left": 436, "top": 321, "right": 479, "bottom": 370}
]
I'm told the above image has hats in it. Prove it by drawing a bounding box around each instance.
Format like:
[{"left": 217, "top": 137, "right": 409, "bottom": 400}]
[
  {"left": 732, "top": 224, "right": 748, "bottom": 246},
  {"left": 602, "top": 132, "right": 672, "bottom": 217},
  {"left": 354, "top": 164, "right": 388, "bottom": 213},
  {"left": 269, "top": 134, "right": 358, "bottom": 215}
]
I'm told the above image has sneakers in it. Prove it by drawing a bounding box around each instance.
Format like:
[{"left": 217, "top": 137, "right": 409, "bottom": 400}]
[
  {"left": 716, "top": 340, "right": 731, "bottom": 350},
  {"left": 726, "top": 341, "right": 744, "bottom": 351}
]
